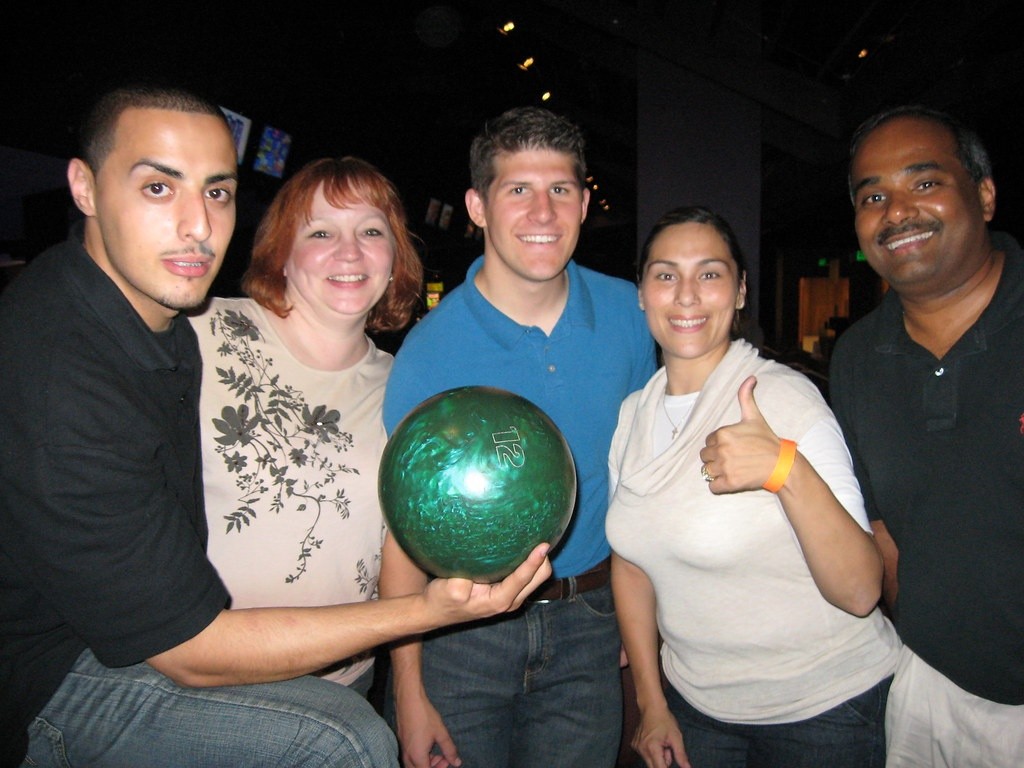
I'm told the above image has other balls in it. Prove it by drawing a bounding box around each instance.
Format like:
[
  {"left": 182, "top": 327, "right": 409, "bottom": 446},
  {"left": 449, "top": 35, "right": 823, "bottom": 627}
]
[{"left": 377, "top": 385, "right": 580, "bottom": 584}]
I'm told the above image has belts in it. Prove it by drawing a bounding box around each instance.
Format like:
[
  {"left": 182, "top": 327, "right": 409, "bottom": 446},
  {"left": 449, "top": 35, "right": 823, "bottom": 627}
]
[{"left": 527, "top": 557, "right": 610, "bottom": 600}]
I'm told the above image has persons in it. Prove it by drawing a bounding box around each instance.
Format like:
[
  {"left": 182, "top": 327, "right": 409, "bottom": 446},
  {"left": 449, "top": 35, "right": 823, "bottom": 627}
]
[
  {"left": 187, "top": 154, "right": 422, "bottom": 702},
  {"left": 376, "top": 105, "right": 658, "bottom": 768},
  {"left": 826, "top": 102, "right": 1024, "bottom": 768},
  {"left": 0, "top": 85, "right": 555, "bottom": 768},
  {"left": 603, "top": 204, "right": 896, "bottom": 768}
]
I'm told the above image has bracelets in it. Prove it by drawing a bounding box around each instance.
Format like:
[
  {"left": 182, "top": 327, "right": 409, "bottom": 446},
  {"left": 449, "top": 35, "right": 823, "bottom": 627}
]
[{"left": 763, "top": 437, "right": 798, "bottom": 495}]
